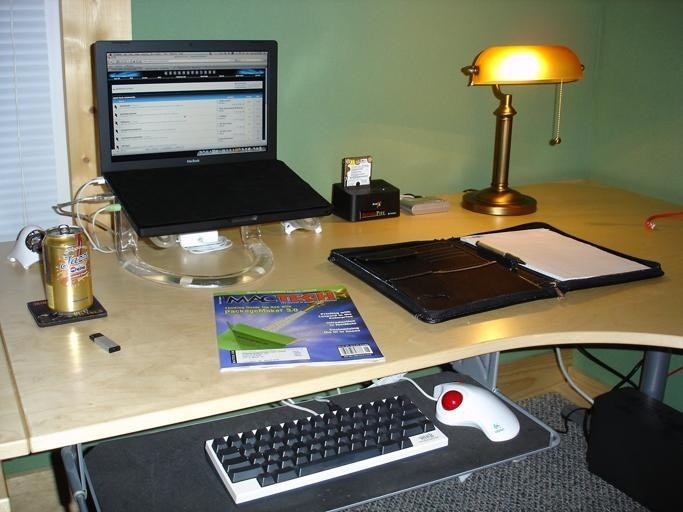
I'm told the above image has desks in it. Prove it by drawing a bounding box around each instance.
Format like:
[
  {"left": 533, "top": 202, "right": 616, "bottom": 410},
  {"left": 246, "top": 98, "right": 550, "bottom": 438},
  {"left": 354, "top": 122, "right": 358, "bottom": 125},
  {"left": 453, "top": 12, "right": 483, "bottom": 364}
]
[{"left": 0, "top": 180, "right": 681, "bottom": 512}]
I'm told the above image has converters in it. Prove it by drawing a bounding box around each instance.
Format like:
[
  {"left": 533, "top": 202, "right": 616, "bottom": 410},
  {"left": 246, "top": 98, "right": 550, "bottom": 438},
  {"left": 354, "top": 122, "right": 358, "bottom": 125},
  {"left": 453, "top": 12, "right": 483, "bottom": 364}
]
[{"left": 177, "top": 230, "right": 219, "bottom": 248}]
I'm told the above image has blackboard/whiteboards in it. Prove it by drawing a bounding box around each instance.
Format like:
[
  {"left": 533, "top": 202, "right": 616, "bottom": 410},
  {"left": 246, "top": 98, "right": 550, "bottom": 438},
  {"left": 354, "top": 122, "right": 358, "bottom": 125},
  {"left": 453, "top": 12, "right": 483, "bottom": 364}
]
[{"left": 0, "top": 0, "right": 74, "bottom": 243}]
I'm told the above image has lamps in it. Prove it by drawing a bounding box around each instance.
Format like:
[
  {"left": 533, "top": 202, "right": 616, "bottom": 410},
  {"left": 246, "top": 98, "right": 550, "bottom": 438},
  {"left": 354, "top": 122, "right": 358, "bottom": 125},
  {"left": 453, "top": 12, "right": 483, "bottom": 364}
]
[{"left": 455, "top": 44, "right": 584, "bottom": 221}]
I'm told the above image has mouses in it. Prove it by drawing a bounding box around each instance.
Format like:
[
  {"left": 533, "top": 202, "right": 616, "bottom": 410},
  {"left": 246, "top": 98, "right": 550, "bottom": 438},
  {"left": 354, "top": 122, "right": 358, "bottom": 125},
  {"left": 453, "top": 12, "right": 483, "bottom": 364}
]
[{"left": 433, "top": 382, "right": 521, "bottom": 443}]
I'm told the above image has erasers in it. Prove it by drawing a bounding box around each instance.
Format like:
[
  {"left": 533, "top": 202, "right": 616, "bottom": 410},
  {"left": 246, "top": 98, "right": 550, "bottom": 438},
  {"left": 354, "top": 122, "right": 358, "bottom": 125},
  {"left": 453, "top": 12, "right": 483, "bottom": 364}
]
[{"left": 90, "top": 333, "right": 120, "bottom": 353}]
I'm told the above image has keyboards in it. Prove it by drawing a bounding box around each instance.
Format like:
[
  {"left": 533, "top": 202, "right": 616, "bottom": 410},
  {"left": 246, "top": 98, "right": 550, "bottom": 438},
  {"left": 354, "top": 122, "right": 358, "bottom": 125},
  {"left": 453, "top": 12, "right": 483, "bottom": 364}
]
[{"left": 204, "top": 393, "right": 449, "bottom": 505}]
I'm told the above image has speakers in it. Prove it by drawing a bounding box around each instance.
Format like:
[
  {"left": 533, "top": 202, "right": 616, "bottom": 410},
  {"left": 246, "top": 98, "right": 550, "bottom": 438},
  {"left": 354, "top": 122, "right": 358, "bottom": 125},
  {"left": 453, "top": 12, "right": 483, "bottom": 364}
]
[
  {"left": 7, "top": 225, "right": 48, "bottom": 272},
  {"left": 279, "top": 217, "right": 323, "bottom": 235}
]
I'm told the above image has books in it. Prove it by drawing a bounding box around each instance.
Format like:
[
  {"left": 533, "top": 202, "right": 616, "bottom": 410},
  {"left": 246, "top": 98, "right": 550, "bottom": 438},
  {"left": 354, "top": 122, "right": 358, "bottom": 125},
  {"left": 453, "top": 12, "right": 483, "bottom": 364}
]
[
  {"left": 211, "top": 282, "right": 387, "bottom": 371},
  {"left": 458, "top": 228, "right": 653, "bottom": 282}
]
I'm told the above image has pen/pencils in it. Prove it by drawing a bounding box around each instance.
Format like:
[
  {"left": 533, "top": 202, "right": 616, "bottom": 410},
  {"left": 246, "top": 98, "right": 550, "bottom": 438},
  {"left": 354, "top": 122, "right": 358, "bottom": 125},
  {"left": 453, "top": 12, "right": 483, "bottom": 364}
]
[{"left": 476, "top": 241, "right": 525, "bottom": 265}]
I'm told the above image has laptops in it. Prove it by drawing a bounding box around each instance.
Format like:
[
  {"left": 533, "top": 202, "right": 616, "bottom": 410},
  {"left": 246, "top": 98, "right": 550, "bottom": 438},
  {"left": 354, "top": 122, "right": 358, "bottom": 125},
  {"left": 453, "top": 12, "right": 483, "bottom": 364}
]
[{"left": 90, "top": 39, "right": 335, "bottom": 238}]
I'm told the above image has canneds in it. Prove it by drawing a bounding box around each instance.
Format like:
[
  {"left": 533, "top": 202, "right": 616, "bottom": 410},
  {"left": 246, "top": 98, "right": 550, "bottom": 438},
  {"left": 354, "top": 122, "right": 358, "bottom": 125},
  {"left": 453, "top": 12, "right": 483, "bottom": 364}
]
[{"left": 42, "top": 224, "right": 92, "bottom": 313}]
